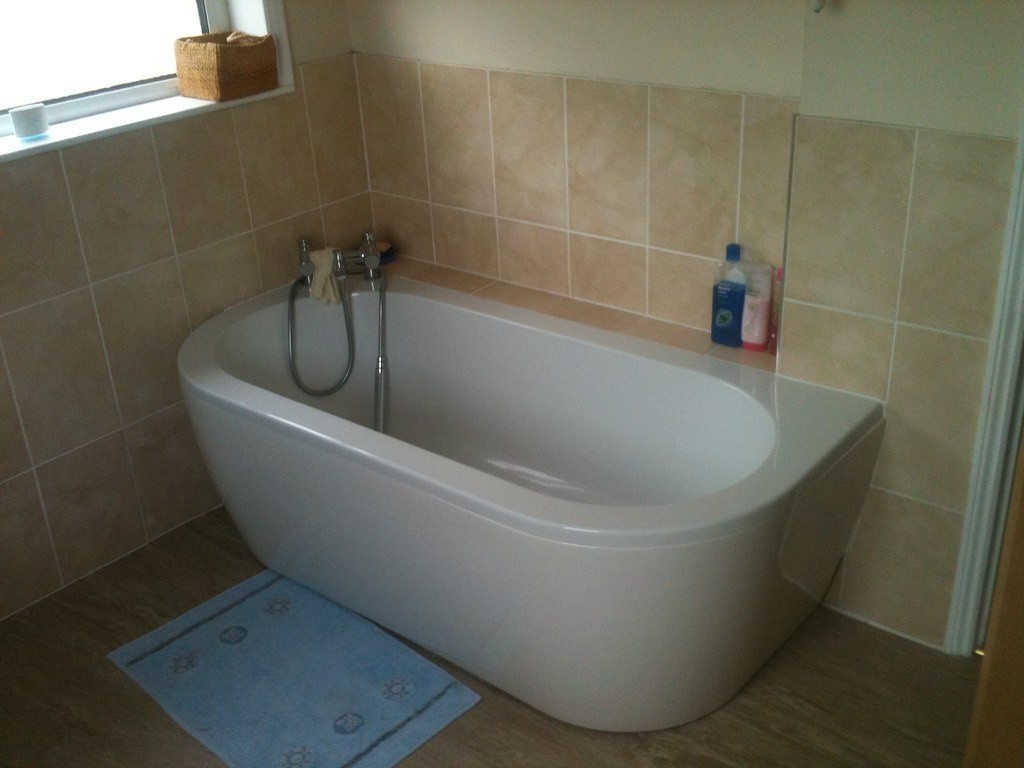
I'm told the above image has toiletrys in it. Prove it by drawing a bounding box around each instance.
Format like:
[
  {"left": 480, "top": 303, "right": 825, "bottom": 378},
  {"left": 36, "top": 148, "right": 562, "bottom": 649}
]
[{"left": 709, "top": 243, "right": 783, "bottom": 357}]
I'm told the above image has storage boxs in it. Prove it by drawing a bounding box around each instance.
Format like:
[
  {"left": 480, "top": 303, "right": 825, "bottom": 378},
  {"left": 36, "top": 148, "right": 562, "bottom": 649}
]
[{"left": 176, "top": 31, "right": 277, "bottom": 102}]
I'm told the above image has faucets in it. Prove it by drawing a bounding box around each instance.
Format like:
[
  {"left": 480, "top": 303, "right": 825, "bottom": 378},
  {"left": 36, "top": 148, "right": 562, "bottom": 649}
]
[{"left": 309, "top": 231, "right": 382, "bottom": 281}]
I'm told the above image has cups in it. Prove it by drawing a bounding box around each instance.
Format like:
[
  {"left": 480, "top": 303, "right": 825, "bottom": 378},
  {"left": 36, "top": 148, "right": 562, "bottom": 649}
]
[{"left": 8, "top": 102, "right": 51, "bottom": 140}]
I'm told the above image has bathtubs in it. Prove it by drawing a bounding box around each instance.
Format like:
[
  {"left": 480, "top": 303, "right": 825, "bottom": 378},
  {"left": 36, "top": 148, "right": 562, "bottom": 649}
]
[{"left": 171, "top": 269, "right": 887, "bottom": 735}]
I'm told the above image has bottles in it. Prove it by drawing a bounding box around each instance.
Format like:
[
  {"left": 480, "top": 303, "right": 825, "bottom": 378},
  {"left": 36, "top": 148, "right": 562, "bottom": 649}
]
[
  {"left": 708, "top": 242, "right": 749, "bottom": 350},
  {"left": 740, "top": 263, "right": 773, "bottom": 351}
]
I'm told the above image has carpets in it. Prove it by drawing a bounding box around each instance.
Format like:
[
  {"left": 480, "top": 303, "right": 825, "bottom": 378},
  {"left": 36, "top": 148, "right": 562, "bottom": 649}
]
[{"left": 107, "top": 569, "right": 483, "bottom": 768}]
